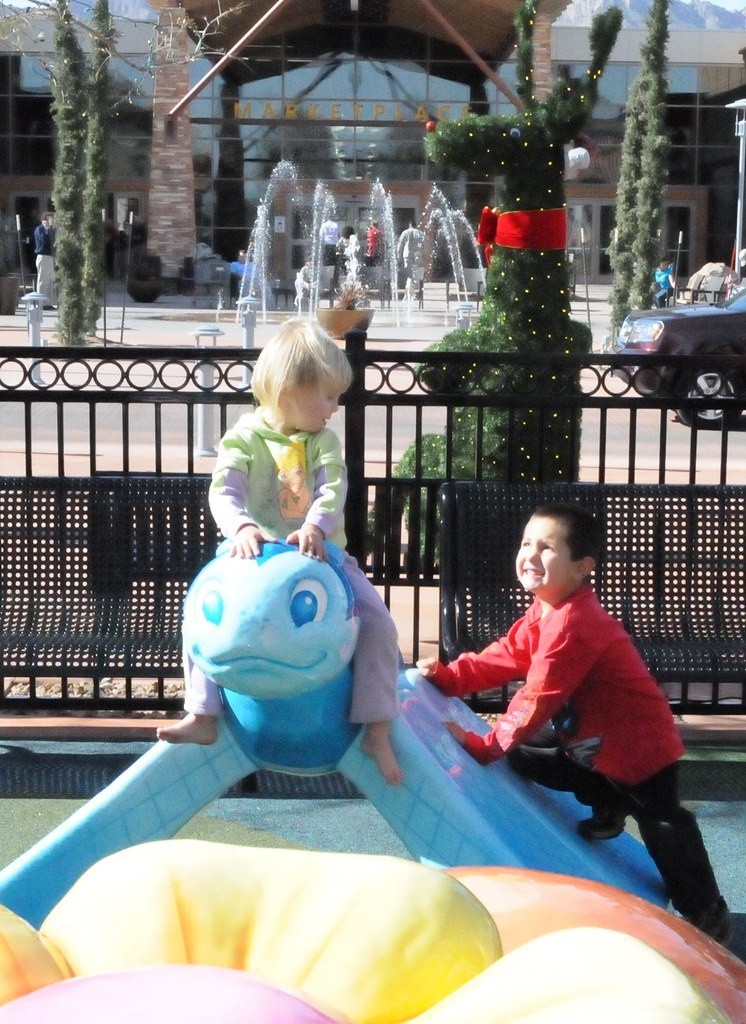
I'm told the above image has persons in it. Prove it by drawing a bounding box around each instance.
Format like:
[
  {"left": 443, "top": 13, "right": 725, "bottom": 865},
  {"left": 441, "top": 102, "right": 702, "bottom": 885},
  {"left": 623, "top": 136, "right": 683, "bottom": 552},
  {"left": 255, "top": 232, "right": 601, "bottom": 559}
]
[
  {"left": 34, "top": 212, "right": 59, "bottom": 310},
  {"left": 230, "top": 249, "right": 273, "bottom": 311},
  {"left": 119, "top": 214, "right": 147, "bottom": 274},
  {"left": 396, "top": 220, "right": 423, "bottom": 267},
  {"left": 653, "top": 257, "right": 675, "bottom": 309},
  {"left": 416, "top": 505, "right": 734, "bottom": 948},
  {"left": 295, "top": 256, "right": 323, "bottom": 301},
  {"left": 318, "top": 215, "right": 381, "bottom": 294},
  {"left": 156, "top": 318, "right": 402, "bottom": 786}
]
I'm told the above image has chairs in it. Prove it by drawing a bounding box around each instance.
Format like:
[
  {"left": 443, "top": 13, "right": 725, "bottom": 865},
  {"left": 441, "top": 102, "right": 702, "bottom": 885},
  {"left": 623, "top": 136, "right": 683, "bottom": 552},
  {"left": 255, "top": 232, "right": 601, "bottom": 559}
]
[
  {"left": 443, "top": 268, "right": 488, "bottom": 309},
  {"left": 665, "top": 274, "right": 705, "bottom": 308},
  {"left": 270, "top": 269, "right": 301, "bottom": 310},
  {"left": 690, "top": 275, "right": 727, "bottom": 306},
  {"left": 388, "top": 266, "right": 426, "bottom": 311},
  {"left": 307, "top": 265, "right": 337, "bottom": 310},
  {"left": 353, "top": 266, "right": 386, "bottom": 309},
  {"left": 191, "top": 263, "right": 234, "bottom": 310}
]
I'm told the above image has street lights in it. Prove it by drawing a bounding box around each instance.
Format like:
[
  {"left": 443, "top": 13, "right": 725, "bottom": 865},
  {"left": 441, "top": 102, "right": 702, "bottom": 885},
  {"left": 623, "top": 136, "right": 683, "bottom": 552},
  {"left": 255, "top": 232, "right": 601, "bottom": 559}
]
[{"left": 723, "top": 99, "right": 746, "bottom": 284}]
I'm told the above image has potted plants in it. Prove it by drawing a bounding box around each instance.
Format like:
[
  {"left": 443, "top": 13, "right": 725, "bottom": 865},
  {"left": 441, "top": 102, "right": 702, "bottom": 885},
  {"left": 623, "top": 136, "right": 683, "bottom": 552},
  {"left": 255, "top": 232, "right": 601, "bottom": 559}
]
[
  {"left": 182, "top": 256, "right": 196, "bottom": 295},
  {"left": 0, "top": 219, "right": 22, "bottom": 316},
  {"left": 126, "top": 249, "right": 165, "bottom": 303},
  {"left": 316, "top": 283, "right": 376, "bottom": 333}
]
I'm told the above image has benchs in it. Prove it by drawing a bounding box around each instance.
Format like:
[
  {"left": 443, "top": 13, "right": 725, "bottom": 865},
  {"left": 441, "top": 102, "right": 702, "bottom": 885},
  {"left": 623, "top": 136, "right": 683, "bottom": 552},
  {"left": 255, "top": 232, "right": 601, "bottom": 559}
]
[
  {"left": 363, "top": 477, "right": 463, "bottom": 586},
  {"left": 433, "top": 478, "right": 746, "bottom": 713},
  {"left": 0, "top": 475, "right": 222, "bottom": 710}
]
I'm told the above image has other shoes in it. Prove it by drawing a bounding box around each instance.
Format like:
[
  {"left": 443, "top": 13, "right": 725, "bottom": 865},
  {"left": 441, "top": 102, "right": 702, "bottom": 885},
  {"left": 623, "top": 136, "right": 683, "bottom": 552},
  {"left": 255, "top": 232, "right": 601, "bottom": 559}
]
[{"left": 43, "top": 306, "right": 56, "bottom": 310}]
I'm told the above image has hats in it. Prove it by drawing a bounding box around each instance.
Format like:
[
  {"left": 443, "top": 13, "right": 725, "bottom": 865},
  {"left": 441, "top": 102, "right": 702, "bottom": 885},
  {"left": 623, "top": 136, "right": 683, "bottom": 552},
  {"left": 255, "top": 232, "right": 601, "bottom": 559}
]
[{"left": 40, "top": 211, "right": 53, "bottom": 220}]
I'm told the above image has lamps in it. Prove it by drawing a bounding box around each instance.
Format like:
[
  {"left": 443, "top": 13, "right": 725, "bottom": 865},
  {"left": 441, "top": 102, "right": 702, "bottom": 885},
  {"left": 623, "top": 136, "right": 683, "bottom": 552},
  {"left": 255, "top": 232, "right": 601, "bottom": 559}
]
[
  {"left": 194, "top": 324, "right": 225, "bottom": 347},
  {"left": 234, "top": 297, "right": 262, "bottom": 328},
  {"left": 21, "top": 291, "right": 47, "bottom": 326},
  {"left": 455, "top": 303, "right": 476, "bottom": 330}
]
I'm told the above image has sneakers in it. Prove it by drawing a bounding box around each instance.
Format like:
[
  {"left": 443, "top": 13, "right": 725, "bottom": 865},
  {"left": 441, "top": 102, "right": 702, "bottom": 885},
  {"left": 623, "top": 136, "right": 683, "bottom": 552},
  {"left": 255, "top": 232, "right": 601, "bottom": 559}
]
[
  {"left": 574, "top": 780, "right": 631, "bottom": 838},
  {"left": 686, "top": 895, "right": 733, "bottom": 947}
]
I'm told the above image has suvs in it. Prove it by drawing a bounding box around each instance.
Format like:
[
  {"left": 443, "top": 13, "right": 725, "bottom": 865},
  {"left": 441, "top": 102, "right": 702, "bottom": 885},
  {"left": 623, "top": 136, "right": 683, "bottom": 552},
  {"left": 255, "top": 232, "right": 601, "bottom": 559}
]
[{"left": 602, "top": 286, "right": 746, "bottom": 431}]
